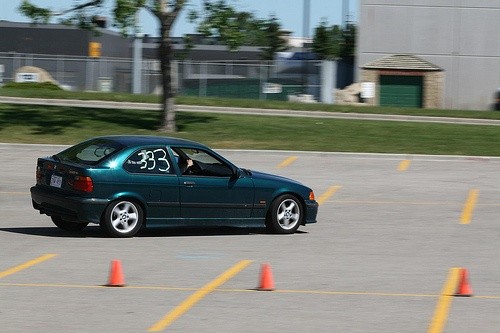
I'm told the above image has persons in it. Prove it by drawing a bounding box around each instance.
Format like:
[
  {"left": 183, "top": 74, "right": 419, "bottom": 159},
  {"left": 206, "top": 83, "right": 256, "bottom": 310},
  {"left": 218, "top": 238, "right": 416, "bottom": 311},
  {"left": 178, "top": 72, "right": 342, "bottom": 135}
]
[{"left": 181, "top": 159, "right": 193, "bottom": 174}]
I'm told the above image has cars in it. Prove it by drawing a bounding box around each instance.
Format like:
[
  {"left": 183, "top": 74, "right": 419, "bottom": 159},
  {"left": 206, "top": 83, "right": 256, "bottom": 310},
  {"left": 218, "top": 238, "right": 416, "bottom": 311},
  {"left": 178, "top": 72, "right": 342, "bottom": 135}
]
[{"left": 30, "top": 135, "right": 318, "bottom": 237}]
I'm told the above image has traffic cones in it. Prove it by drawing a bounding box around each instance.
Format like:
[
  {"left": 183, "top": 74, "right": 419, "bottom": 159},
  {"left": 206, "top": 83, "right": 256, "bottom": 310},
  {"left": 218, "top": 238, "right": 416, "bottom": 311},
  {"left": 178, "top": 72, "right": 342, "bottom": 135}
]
[
  {"left": 253, "top": 263, "right": 275, "bottom": 291},
  {"left": 104, "top": 259, "right": 126, "bottom": 287},
  {"left": 453, "top": 268, "right": 473, "bottom": 297}
]
